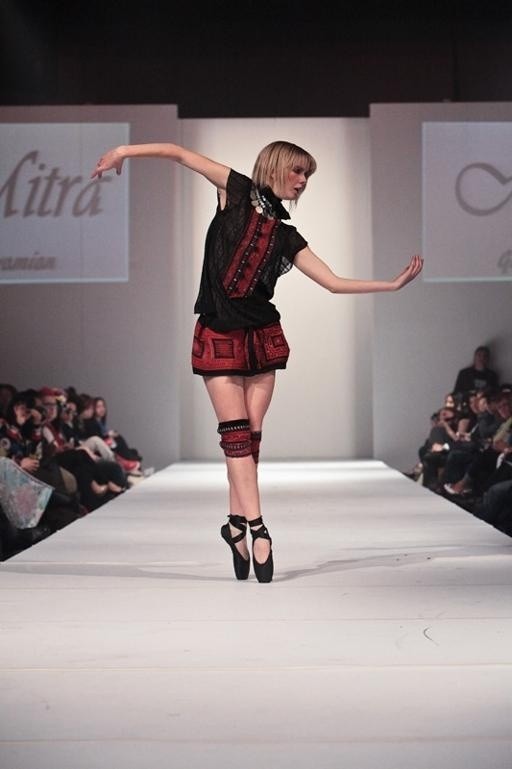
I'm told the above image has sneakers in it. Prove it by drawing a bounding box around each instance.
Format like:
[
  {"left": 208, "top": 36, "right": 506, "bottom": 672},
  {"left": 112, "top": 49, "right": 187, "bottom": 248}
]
[
  {"left": 444, "top": 482, "right": 460, "bottom": 495},
  {"left": 116, "top": 455, "right": 140, "bottom": 472},
  {"left": 221, "top": 514, "right": 250, "bottom": 580},
  {"left": 249, "top": 516, "right": 274, "bottom": 583}
]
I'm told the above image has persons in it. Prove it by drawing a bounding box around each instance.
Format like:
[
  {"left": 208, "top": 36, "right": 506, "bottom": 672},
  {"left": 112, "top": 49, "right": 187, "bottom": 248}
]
[
  {"left": 90, "top": 139, "right": 426, "bottom": 583},
  {"left": 414, "top": 346, "right": 512, "bottom": 540},
  {"left": 0, "top": 382, "right": 143, "bottom": 555}
]
